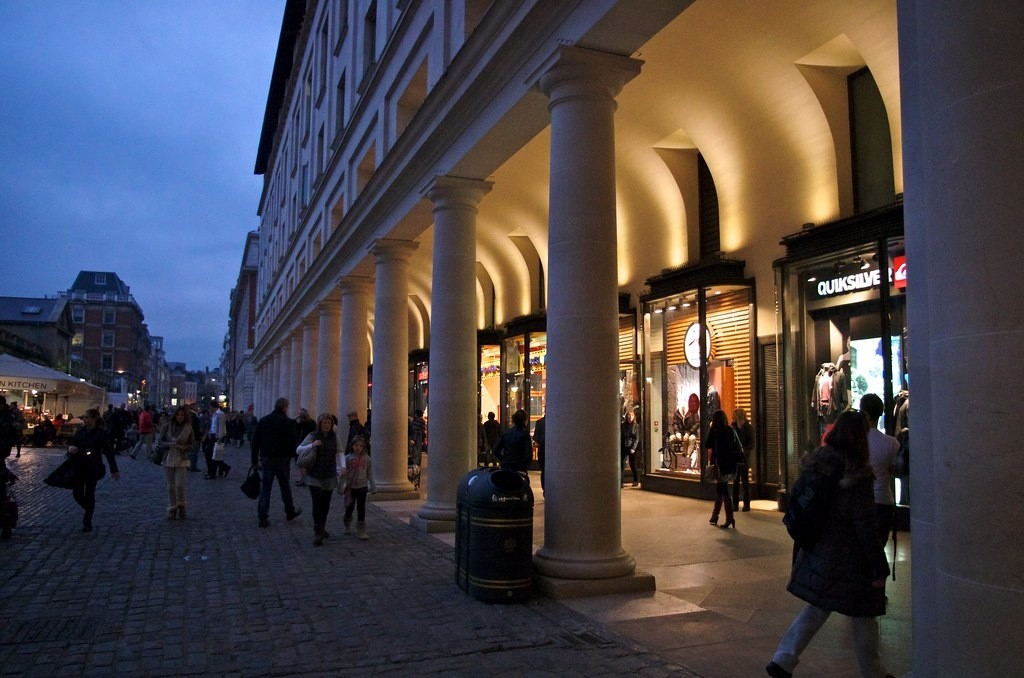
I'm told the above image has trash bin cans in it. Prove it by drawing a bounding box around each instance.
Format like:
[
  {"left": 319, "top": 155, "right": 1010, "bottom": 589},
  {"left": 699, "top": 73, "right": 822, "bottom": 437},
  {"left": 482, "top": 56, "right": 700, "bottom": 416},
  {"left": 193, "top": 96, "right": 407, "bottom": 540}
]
[{"left": 454, "top": 466, "right": 534, "bottom": 604}]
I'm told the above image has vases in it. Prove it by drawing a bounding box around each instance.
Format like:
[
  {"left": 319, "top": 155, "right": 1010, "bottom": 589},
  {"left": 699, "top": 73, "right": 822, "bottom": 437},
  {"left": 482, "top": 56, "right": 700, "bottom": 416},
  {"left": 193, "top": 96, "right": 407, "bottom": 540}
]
[{"left": 677, "top": 458, "right": 692, "bottom": 470}]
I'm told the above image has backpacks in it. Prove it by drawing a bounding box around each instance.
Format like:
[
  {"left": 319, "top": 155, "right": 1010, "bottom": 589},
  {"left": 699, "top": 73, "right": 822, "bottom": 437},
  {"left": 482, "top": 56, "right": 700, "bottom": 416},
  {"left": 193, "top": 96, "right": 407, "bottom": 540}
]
[{"left": 780, "top": 455, "right": 848, "bottom": 544}]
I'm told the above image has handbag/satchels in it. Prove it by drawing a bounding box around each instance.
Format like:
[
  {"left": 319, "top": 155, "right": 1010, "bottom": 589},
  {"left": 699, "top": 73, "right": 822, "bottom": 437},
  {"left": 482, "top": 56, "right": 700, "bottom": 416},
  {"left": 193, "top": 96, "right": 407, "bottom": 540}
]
[
  {"left": 240, "top": 465, "right": 261, "bottom": 499},
  {"left": 152, "top": 445, "right": 164, "bottom": 467},
  {"left": 705, "top": 464, "right": 721, "bottom": 480},
  {"left": 296, "top": 433, "right": 315, "bottom": 467}
]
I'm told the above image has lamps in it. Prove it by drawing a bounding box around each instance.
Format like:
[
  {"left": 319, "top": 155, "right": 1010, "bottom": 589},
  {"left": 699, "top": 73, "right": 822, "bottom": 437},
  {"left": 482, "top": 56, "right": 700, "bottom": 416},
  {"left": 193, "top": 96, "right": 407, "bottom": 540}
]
[
  {"left": 679, "top": 295, "right": 691, "bottom": 308},
  {"left": 654, "top": 302, "right": 663, "bottom": 314},
  {"left": 667, "top": 299, "right": 676, "bottom": 310},
  {"left": 530, "top": 338, "right": 533, "bottom": 343},
  {"left": 804, "top": 271, "right": 817, "bottom": 281},
  {"left": 694, "top": 293, "right": 708, "bottom": 304},
  {"left": 834, "top": 264, "right": 847, "bottom": 278},
  {"left": 860, "top": 258, "right": 870, "bottom": 270}
]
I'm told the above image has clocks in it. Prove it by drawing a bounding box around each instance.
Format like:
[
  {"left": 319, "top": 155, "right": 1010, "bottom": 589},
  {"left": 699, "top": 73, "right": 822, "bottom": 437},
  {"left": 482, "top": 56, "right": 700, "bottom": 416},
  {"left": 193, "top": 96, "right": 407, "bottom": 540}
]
[{"left": 683, "top": 319, "right": 716, "bottom": 371}]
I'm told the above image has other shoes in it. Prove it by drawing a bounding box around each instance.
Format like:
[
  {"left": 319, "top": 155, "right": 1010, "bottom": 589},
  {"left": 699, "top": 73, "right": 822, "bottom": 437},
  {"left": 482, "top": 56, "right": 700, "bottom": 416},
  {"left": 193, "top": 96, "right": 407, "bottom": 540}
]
[
  {"left": 167, "top": 506, "right": 175, "bottom": 519},
  {"left": 632, "top": 481, "right": 638, "bottom": 487},
  {"left": 81, "top": 525, "right": 94, "bottom": 532},
  {"left": 259, "top": 520, "right": 271, "bottom": 528},
  {"left": 313, "top": 534, "right": 324, "bottom": 546},
  {"left": 287, "top": 507, "right": 303, "bottom": 521},
  {"left": 766, "top": 662, "right": 793, "bottom": 678},
  {"left": 295, "top": 479, "right": 306, "bottom": 488},
  {"left": 188, "top": 464, "right": 231, "bottom": 480},
  {"left": 129, "top": 454, "right": 137, "bottom": 460},
  {"left": 323, "top": 530, "right": 329, "bottom": 539},
  {"left": 179, "top": 504, "right": 186, "bottom": 518}
]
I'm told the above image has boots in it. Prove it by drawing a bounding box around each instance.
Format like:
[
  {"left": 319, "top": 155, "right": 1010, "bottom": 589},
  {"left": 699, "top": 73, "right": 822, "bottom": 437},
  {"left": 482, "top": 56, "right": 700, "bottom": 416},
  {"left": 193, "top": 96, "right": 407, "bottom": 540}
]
[
  {"left": 709, "top": 503, "right": 722, "bottom": 525},
  {"left": 344, "top": 517, "right": 351, "bottom": 535},
  {"left": 732, "top": 499, "right": 739, "bottom": 512},
  {"left": 356, "top": 521, "right": 369, "bottom": 539},
  {"left": 742, "top": 499, "right": 750, "bottom": 512},
  {"left": 720, "top": 507, "right": 736, "bottom": 529}
]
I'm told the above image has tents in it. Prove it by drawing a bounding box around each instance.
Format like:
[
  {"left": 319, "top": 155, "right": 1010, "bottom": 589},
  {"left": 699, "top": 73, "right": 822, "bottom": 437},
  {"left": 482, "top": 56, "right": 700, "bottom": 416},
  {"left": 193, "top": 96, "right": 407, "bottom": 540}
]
[{"left": 0, "top": 352, "right": 128, "bottom": 418}]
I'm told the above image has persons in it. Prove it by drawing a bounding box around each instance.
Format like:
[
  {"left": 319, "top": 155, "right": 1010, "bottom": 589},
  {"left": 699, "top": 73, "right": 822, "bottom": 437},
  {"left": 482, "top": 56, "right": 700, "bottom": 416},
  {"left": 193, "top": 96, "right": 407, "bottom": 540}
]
[
  {"left": 295, "top": 408, "right": 317, "bottom": 487},
  {"left": 836, "top": 335, "right": 851, "bottom": 406},
  {"left": 65, "top": 409, "right": 121, "bottom": 532},
  {"left": 810, "top": 363, "right": 848, "bottom": 437},
  {"left": 766, "top": 411, "right": 894, "bottom": 678},
  {"left": 705, "top": 410, "right": 738, "bottom": 529},
  {"left": 669, "top": 385, "right": 721, "bottom": 468},
  {"left": 330, "top": 409, "right": 425, "bottom": 495},
  {"left": 494, "top": 409, "right": 533, "bottom": 475},
  {"left": 731, "top": 407, "right": 755, "bottom": 512},
  {"left": 532, "top": 405, "right": 546, "bottom": 498},
  {"left": 295, "top": 412, "right": 347, "bottom": 546},
  {"left": 620, "top": 410, "right": 640, "bottom": 488},
  {"left": 860, "top": 394, "right": 900, "bottom": 603},
  {"left": 0, "top": 394, "right": 39, "bottom": 484},
  {"left": 477, "top": 411, "right": 503, "bottom": 468},
  {"left": 157, "top": 405, "right": 196, "bottom": 519},
  {"left": 39, "top": 399, "right": 260, "bottom": 479},
  {"left": 339, "top": 435, "right": 377, "bottom": 538},
  {"left": 250, "top": 397, "right": 300, "bottom": 529}
]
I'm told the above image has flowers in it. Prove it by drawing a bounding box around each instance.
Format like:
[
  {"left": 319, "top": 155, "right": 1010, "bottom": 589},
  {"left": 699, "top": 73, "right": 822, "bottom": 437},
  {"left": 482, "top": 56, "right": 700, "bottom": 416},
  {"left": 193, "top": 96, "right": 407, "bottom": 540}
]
[{"left": 668, "top": 433, "right": 698, "bottom": 458}]
